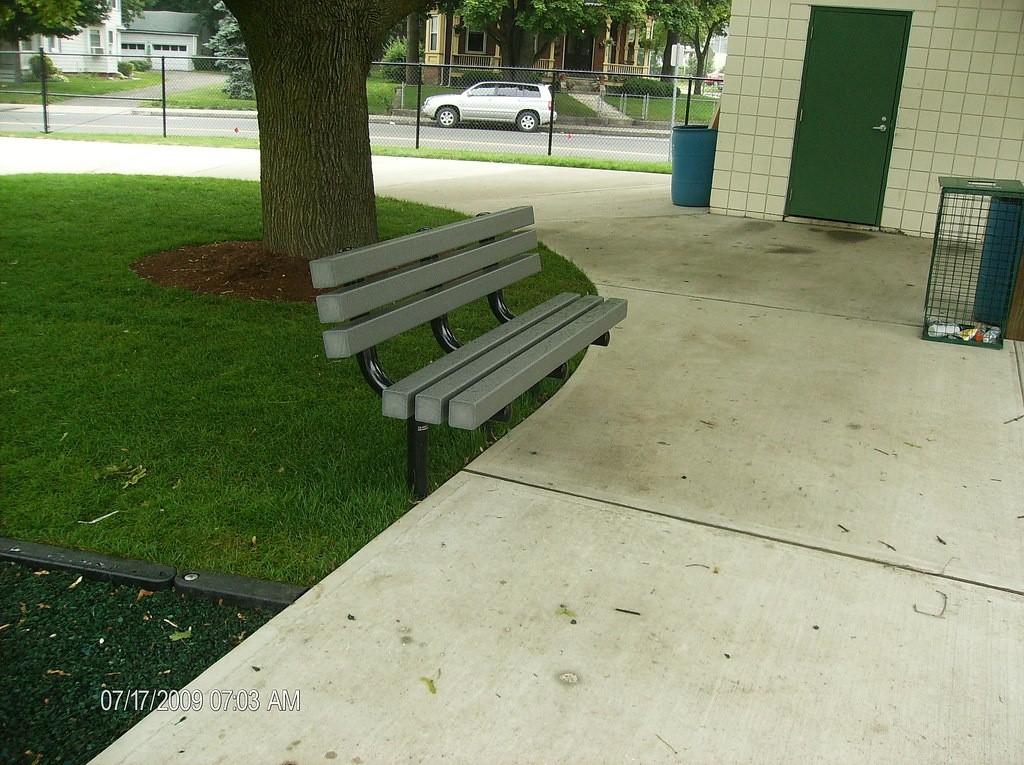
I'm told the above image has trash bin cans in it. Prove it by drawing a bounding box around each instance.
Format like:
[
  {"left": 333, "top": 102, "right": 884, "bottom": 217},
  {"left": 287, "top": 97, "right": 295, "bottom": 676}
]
[
  {"left": 670, "top": 125, "right": 718, "bottom": 207},
  {"left": 921, "top": 176, "right": 1024, "bottom": 351}
]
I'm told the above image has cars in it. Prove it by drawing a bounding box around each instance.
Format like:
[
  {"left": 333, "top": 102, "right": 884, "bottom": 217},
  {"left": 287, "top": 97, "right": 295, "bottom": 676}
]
[{"left": 707, "top": 73, "right": 723, "bottom": 86}]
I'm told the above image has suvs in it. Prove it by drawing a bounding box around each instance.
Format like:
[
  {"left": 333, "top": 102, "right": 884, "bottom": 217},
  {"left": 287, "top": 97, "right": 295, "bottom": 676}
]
[{"left": 423, "top": 81, "right": 557, "bottom": 133}]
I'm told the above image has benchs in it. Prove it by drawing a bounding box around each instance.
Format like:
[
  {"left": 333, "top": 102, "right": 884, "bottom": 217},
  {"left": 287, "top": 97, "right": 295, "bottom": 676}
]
[{"left": 308, "top": 205, "right": 628, "bottom": 503}]
